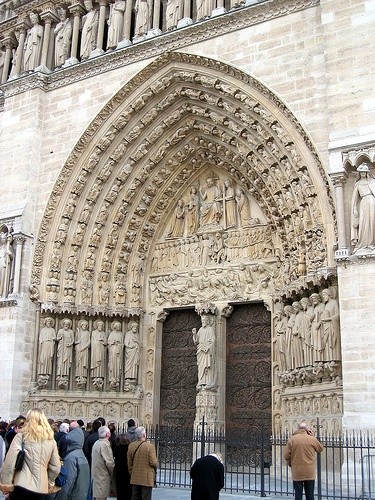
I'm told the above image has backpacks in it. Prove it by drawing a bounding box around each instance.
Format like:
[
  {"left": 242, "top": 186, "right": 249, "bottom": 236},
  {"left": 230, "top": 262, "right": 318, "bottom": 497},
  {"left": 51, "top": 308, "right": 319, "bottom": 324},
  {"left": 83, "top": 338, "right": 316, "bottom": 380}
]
[{"left": 55, "top": 467, "right": 68, "bottom": 487}]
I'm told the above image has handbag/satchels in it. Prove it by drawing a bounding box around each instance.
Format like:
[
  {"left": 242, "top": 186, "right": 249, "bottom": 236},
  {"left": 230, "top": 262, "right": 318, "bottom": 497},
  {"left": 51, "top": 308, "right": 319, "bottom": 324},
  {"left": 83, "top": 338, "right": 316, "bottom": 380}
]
[{"left": 15, "top": 433, "right": 25, "bottom": 470}]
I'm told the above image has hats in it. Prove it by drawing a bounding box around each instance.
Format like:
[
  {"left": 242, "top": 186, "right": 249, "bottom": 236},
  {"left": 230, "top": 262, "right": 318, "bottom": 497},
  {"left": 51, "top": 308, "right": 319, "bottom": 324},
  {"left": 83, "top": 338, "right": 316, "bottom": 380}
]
[
  {"left": 107, "top": 420, "right": 117, "bottom": 425},
  {"left": 16, "top": 419, "right": 26, "bottom": 425}
]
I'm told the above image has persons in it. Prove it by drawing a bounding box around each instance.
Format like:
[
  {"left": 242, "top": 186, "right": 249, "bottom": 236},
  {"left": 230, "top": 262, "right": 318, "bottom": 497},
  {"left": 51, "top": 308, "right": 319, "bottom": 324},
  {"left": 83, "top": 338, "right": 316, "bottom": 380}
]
[
  {"left": 0, "top": 408, "right": 61, "bottom": 500},
  {"left": 189, "top": 452, "right": 224, "bottom": 500},
  {"left": 284, "top": 423, "right": 324, "bottom": 500},
  {"left": 0, "top": 415, "right": 159, "bottom": 500}
]
